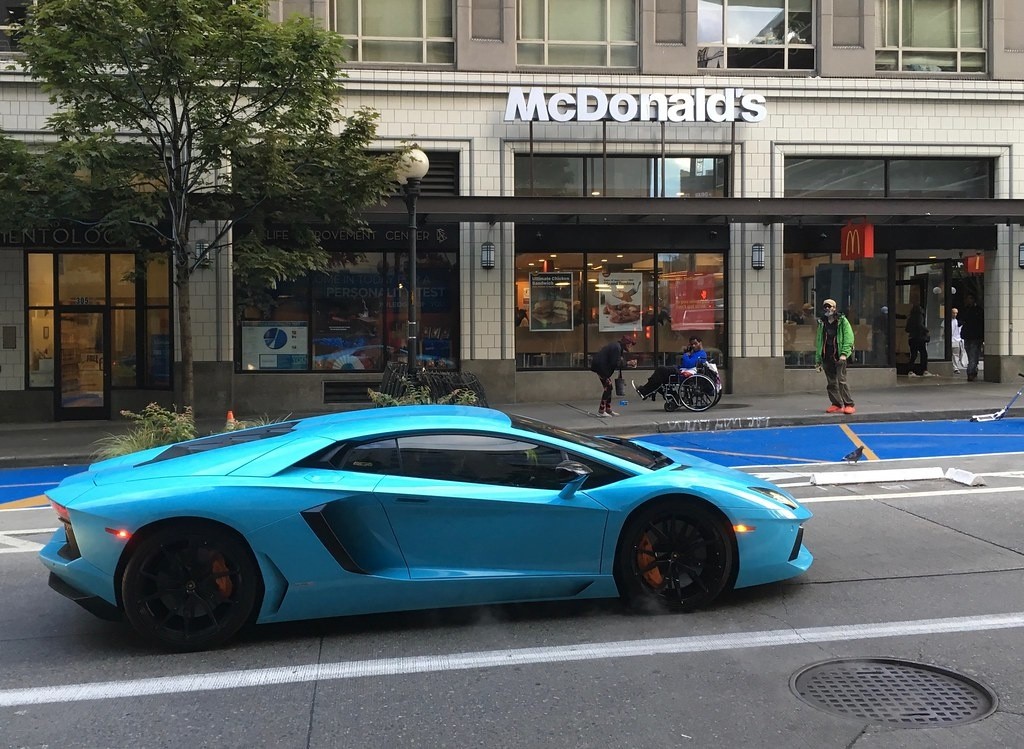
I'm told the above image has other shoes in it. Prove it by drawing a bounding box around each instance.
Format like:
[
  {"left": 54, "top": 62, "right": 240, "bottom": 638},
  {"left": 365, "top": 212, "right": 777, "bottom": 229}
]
[
  {"left": 923, "top": 371, "right": 933, "bottom": 377},
  {"left": 954, "top": 369, "right": 960, "bottom": 374},
  {"left": 826, "top": 405, "right": 844, "bottom": 413},
  {"left": 844, "top": 406, "right": 855, "bottom": 414},
  {"left": 631, "top": 380, "right": 645, "bottom": 398},
  {"left": 606, "top": 410, "right": 620, "bottom": 417},
  {"left": 908, "top": 372, "right": 920, "bottom": 378},
  {"left": 597, "top": 411, "right": 612, "bottom": 417}
]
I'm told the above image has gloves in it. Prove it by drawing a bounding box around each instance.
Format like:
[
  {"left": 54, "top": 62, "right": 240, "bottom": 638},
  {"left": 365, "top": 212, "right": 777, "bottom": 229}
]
[{"left": 815, "top": 363, "right": 824, "bottom": 374}]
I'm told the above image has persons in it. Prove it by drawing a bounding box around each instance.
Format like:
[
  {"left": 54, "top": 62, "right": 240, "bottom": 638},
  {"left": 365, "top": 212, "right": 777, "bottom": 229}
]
[
  {"left": 801, "top": 303, "right": 815, "bottom": 325},
  {"left": 590, "top": 335, "right": 637, "bottom": 418},
  {"left": 783, "top": 302, "right": 803, "bottom": 326},
  {"left": 872, "top": 305, "right": 909, "bottom": 370},
  {"left": 904, "top": 304, "right": 930, "bottom": 372},
  {"left": 939, "top": 308, "right": 962, "bottom": 375},
  {"left": 814, "top": 300, "right": 856, "bottom": 414},
  {"left": 516, "top": 307, "right": 528, "bottom": 328},
  {"left": 956, "top": 293, "right": 985, "bottom": 383},
  {"left": 632, "top": 336, "right": 707, "bottom": 400}
]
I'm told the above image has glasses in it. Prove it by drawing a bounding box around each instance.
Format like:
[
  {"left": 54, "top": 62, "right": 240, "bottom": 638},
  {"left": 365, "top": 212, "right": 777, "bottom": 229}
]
[{"left": 824, "top": 305, "right": 832, "bottom": 309}]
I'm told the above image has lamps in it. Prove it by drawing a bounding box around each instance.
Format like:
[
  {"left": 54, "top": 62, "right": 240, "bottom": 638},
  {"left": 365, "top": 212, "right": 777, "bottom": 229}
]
[
  {"left": 1019, "top": 243, "right": 1024, "bottom": 269},
  {"left": 752, "top": 243, "right": 765, "bottom": 270},
  {"left": 195, "top": 239, "right": 209, "bottom": 266},
  {"left": 481, "top": 242, "right": 495, "bottom": 270}
]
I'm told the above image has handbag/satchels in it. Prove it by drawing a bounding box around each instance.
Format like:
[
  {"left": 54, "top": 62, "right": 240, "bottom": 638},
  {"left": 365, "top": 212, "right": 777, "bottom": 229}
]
[
  {"left": 614, "top": 369, "right": 626, "bottom": 396},
  {"left": 961, "top": 348, "right": 969, "bottom": 367}
]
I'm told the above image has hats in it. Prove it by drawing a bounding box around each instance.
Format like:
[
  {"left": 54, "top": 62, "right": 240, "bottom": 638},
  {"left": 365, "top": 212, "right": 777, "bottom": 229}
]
[
  {"left": 621, "top": 335, "right": 636, "bottom": 345},
  {"left": 823, "top": 299, "right": 836, "bottom": 306}
]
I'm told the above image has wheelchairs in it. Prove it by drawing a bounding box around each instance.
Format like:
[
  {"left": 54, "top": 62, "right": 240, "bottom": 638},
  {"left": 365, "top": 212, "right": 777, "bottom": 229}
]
[{"left": 636, "top": 357, "right": 723, "bottom": 412}]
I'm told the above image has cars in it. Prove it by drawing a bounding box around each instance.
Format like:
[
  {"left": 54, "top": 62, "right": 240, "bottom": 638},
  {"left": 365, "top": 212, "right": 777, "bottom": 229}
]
[{"left": 314, "top": 344, "right": 456, "bottom": 372}]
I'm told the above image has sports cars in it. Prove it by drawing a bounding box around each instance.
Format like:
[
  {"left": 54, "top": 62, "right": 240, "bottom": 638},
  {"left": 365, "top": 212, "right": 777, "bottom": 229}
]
[{"left": 39, "top": 403, "right": 814, "bottom": 653}]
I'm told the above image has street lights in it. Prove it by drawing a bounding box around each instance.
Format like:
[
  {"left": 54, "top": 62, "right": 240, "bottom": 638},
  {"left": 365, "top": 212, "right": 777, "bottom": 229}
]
[{"left": 394, "top": 149, "right": 430, "bottom": 379}]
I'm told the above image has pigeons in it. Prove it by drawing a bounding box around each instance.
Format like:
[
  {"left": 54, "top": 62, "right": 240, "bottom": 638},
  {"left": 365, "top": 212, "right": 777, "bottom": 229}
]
[{"left": 841, "top": 446, "right": 865, "bottom": 466}]
